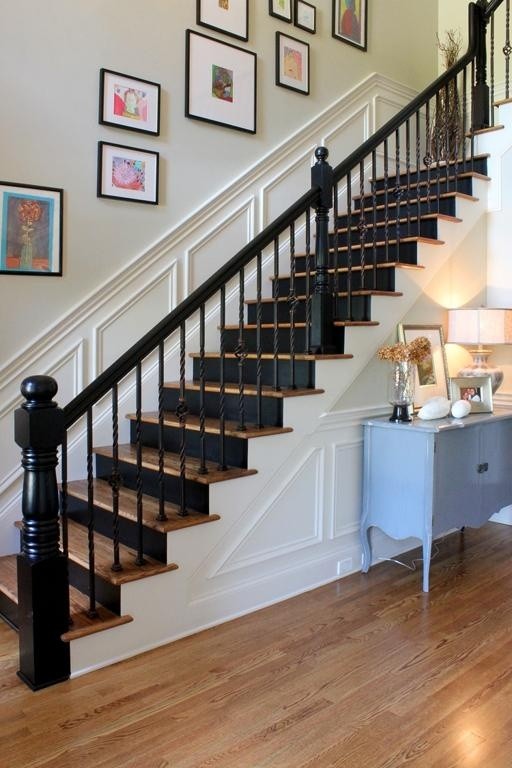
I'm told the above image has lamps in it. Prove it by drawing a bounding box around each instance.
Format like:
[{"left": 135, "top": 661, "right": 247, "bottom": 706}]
[{"left": 444, "top": 307, "right": 512, "bottom": 396}]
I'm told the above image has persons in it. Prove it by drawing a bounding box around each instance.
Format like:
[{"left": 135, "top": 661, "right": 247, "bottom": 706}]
[
  {"left": 461, "top": 390, "right": 468, "bottom": 400},
  {"left": 470, "top": 387, "right": 480, "bottom": 401},
  {"left": 467, "top": 388, "right": 473, "bottom": 401}
]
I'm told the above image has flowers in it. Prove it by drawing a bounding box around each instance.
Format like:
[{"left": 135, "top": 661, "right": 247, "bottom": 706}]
[{"left": 376, "top": 337, "right": 433, "bottom": 404}]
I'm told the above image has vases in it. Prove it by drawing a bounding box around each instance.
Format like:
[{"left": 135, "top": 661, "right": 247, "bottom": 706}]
[
  {"left": 430, "top": 78, "right": 462, "bottom": 164},
  {"left": 387, "top": 361, "right": 416, "bottom": 425}
]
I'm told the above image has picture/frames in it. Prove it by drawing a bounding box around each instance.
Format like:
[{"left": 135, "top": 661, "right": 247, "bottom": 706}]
[
  {"left": 396, "top": 322, "right": 450, "bottom": 415},
  {"left": 447, "top": 375, "right": 495, "bottom": 416},
  {"left": 0, "top": 180, "right": 64, "bottom": 281},
  {"left": 183, "top": 0, "right": 368, "bottom": 135},
  {"left": 95, "top": 66, "right": 161, "bottom": 205}
]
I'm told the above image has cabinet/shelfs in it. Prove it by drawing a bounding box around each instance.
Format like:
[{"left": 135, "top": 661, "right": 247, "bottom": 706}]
[{"left": 359, "top": 410, "right": 512, "bottom": 596}]
